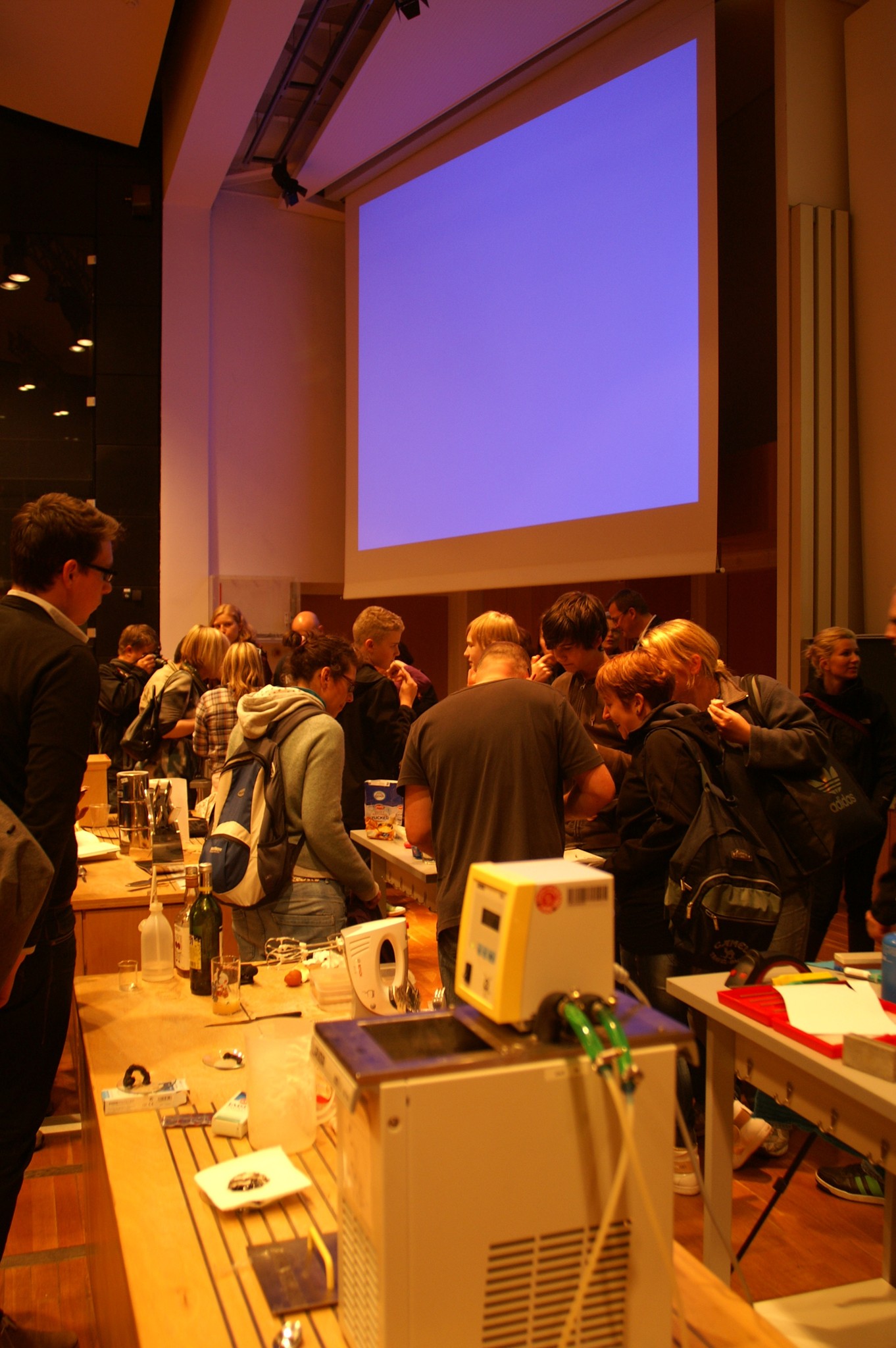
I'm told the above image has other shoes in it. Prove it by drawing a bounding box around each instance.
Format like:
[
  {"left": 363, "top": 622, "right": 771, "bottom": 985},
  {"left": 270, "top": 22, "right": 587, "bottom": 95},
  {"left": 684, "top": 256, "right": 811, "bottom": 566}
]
[{"left": 0, "top": 1315, "right": 78, "bottom": 1348}]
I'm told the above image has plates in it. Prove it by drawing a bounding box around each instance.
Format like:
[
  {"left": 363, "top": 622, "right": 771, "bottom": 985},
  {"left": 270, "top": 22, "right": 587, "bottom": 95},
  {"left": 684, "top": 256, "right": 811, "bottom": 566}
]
[{"left": 201, "top": 1046, "right": 247, "bottom": 1069}]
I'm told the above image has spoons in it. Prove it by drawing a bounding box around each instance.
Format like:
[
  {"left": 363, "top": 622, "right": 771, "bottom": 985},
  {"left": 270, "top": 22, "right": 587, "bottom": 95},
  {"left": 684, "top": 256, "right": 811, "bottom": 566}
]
[{"left": 78, "top": 865, "right": 87, "bottom": 883}]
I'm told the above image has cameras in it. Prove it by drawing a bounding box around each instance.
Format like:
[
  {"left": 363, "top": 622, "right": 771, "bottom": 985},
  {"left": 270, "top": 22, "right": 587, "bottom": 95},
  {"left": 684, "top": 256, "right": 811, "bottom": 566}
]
[{"left": 152, "top": 654, "right": 166, "bottom": 670}]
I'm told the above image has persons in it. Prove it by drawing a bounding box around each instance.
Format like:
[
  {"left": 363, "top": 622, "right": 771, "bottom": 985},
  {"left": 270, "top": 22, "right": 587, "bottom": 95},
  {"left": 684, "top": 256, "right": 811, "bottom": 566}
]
[
  {"left": 0, "top": 492, "right": 118, "bottom": 1348},
  {"left": 395, "top": 640, "right": 615, "bottom": 1011},
  {"left": 310, "top": 605, "right": 418, "bottom": 929},
  {"left": 97, "top": 591, "right": 896, "bottom": 1200},
  {"left": 193, "top": 640, "right": 270, "bottom": 807}
]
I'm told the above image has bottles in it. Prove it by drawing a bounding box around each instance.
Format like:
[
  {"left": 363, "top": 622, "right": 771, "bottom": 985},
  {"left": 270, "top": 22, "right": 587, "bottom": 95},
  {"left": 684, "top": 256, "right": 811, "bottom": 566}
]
[
  {"left": 189, "top": 862, "right": 223, "bottom": 995},
  {"left": 174, "top": 866, "right": 199, "bottom": 978},
  {"left": 140, "top": 900, "right": 174, "bottom": 981}
]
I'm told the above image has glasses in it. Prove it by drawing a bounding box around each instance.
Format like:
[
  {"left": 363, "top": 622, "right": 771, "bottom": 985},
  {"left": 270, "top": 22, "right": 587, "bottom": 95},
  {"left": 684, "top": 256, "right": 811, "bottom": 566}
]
[
  {"left": 57, "top": 560, "right": 118, "bottom": 582},
  {"left": 337, "top": 673, "right": 359, "bottom": 693},
  {"left": 612, "top": 609, "right": 628, "bottom": 624}
]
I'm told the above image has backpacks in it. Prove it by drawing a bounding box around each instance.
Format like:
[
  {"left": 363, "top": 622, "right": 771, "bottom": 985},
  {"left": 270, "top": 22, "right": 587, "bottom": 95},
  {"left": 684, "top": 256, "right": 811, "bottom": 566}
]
[
  {"left": 199, "top": 702, "right": 329, "bottom": 910},
  {"left": 642, "top": 726, "right": 784, "bottom": 974}
]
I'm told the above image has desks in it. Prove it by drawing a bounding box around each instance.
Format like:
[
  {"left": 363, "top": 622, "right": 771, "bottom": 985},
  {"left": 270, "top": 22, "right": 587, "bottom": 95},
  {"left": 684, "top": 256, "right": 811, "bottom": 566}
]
[
  {"left": 665, "top": 950, "right": 896, "bottom": 1287},
  {"left": 72, "top": 957, "right": 796, "bottom": 1348},
  {"left": 70, "top": 810, "right": 206, "bottom": 973},
  {"left": 350, "top": 823, "right": 605, "bottom": 914}
]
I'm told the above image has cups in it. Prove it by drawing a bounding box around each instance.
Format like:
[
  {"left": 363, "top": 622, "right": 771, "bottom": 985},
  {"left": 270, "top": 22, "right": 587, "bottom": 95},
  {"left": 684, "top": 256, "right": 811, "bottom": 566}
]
[
  {"left": 327, "top": 933, "right": 346, "bottom": 968},
  {"left": 118, "top": 960, "right": 138, "bottom": 992},
  {"left": 210, "top": 955, "right": 241, "bottom": 1016},
  {"left": 247, "top": 1044, "right": 338, "bottom": 1154},
  {"left": 89, "top": 803, "right": 111, "bottom": 829}
]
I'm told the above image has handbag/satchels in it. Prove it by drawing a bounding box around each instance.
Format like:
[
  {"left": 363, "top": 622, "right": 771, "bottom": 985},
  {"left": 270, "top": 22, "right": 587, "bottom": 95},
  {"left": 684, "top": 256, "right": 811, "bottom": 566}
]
[
  {"left": 746, "top": 673, "right": 882, "bottom": 877},
  {"left": 119, "top": 669, "right": 193, "bottom": 762}
]
